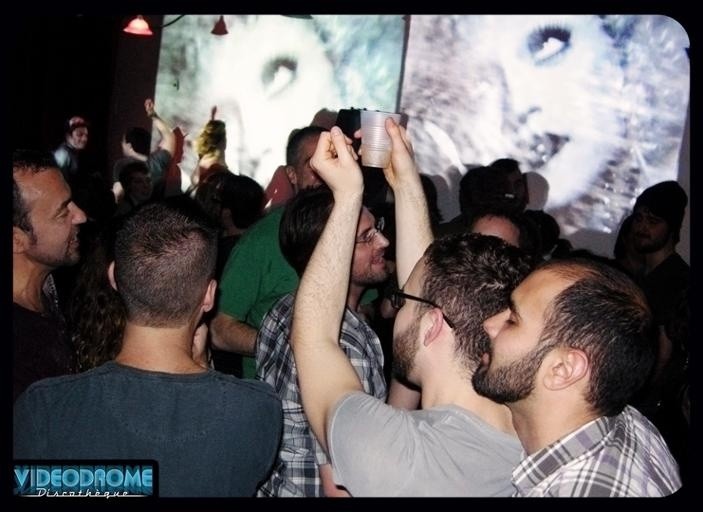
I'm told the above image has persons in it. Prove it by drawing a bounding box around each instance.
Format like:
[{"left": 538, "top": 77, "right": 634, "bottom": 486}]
[
  {"left": 446, "top": 13, "right": 645, "bottom": 242},
  {"left": 12, "top": 97, "right": 692, "bottom": 500},
  {"left": 193, "top": 14, "right": 353, "bottom": 209}
]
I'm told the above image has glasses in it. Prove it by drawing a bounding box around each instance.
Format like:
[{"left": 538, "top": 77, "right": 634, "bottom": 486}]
[
  {"left": 355, "top": 216, "right": 385, "bottom": 244},
  {"left": 388, "top": 286, "right": 454, "bottom": 328}
]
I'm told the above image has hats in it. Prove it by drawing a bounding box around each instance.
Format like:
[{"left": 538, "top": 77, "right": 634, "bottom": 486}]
[{"left": 636, "top": 181, "right": 688, "bottom": 224}]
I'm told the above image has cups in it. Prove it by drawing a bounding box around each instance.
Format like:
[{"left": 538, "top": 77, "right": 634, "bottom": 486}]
[{"left": 358, "top": 109, "right": 401, "bottom": 171}]
